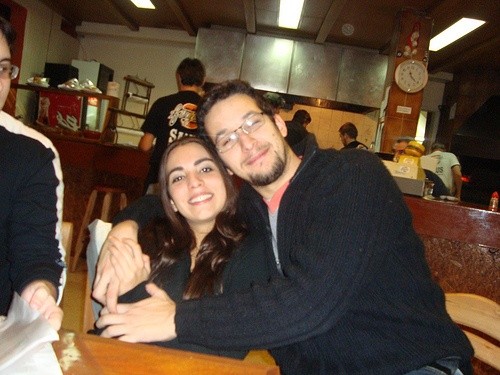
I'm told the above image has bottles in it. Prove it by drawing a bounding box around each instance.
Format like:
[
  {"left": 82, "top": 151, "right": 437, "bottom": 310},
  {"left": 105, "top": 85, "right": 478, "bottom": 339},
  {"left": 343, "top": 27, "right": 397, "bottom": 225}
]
[{"left": 489, "top": 191, "right": 499, "bottom": 212}]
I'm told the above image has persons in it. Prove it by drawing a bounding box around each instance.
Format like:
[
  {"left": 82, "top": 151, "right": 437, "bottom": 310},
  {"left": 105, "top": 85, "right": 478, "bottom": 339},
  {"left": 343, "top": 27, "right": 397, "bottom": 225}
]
[
  {"left": 91, "top": 80, "right": 475, "bottom": 375},
  {"left": 138, "top": 58, "right": 207, "bottom": 195},
  {"left": 261, "top": 91, "right": 463, "bottom": 202},
  {"left": 0, "top": 16, "right": 66, "bottom": 345},
  {"left": 87, "top": 137, "right": 271, "bottom": 360}
]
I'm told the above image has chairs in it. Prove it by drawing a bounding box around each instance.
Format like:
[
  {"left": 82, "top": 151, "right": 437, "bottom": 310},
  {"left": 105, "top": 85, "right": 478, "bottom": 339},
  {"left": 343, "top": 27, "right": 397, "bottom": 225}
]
[{"left": 445, "top": 292, "right": 500, "bottom": 371}]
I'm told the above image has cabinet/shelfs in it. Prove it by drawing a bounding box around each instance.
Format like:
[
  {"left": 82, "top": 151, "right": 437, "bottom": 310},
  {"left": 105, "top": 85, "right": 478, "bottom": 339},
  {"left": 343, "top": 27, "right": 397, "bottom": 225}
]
[
  {"left": 193, "top": 26, "right": 390, "bottom": 114},
  {"left": 26, "top": 84, "right": 120, "bottom": 143}
]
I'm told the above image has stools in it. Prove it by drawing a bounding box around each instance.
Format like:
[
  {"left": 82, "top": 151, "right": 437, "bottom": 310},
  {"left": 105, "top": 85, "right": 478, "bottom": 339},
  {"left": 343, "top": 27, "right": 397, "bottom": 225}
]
[{"left": 71, "top": 184, "right": 127, "bottom": 272}]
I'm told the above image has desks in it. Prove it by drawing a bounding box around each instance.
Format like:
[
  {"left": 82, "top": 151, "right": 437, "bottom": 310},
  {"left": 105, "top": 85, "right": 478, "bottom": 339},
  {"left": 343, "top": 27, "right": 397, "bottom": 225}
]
[{"left": 52, "top": 328, "right": 280, "bottom": 375}]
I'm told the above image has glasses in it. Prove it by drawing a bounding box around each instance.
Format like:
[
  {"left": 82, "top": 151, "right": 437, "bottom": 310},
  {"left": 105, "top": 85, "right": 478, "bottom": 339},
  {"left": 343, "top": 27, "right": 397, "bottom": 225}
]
[
  {"left": 0, "top": 64, "right": 19, "bottom": 79},
  {"left": 215, "top": 112, "right": 265, "bottom": 153}
]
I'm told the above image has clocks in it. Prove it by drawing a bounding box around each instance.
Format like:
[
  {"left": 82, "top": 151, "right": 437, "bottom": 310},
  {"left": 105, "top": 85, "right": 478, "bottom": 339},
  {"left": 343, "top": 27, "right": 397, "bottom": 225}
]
[{"left": 395, "top": 60, "right": 428, "bottom": 94}]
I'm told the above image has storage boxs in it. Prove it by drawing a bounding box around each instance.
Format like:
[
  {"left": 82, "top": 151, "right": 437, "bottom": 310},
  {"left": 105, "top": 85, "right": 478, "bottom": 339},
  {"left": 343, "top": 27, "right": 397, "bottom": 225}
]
[{"left": 393, "top": 176, "right": 425, "bottom": 196}]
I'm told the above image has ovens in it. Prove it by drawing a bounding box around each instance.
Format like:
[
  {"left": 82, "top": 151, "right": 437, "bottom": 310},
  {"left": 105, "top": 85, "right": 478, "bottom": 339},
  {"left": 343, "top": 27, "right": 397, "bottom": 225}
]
[{"left": 33, "top": 89, "right": 120, "bottom": 142}]
